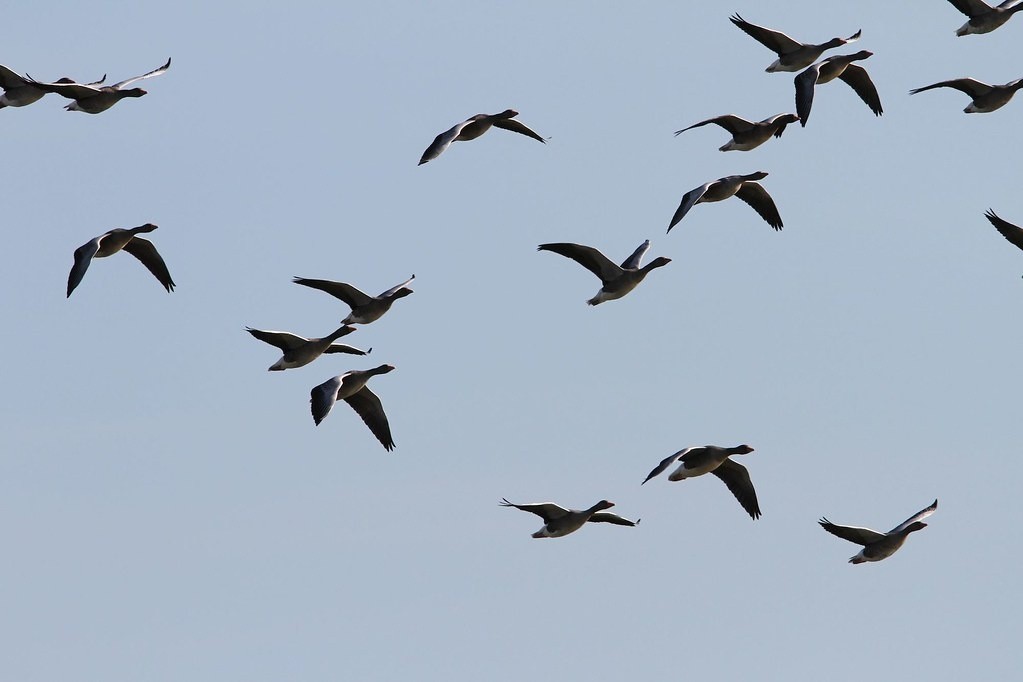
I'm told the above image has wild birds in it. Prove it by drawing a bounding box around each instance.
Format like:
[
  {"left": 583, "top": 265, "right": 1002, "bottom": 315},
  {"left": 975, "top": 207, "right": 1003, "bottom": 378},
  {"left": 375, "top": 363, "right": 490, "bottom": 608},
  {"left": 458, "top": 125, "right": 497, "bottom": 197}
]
[
  {"left": 676, "top": 113, "right": 802, "bottom": 153},
  {"left": 727, "top": 12, "right": 864, "bottom": 74},
  {"left": 0, "top": 64, "right": 77, "bottom": 108},
  {"left": 417, "top": 109, "right": 549, "bottom": 167},
  {"left": 26, "top": 55, "right": 172, "bottom": 114},
  {"left": 947, "top": 0, "right": 1023, "bottom": 38},
  {"left": 819, "top": 499, "right": 940, "bottom": 564},
  {"left": 497, "top": 498, "right": 641, "bottom": 538},
  {"left": 292, "top": 273, "right": 415, "bottom": 326},
  {"left": 909, "top": 79, "right": 1023, "bottom": 114},
  {"left": 983, "top": 208, "right": 1023, "bottom": 279},
  {"left": 66, "top": 222, "right": 176, "bottom": 294},
  {"left": 244, "top": 324, "right": 375, "bottom": 372},
  {"left": 309, "top": 364, "right": 398, "bottom": 453},
  {"left": 666, "top": 170, "right": 785, "bottom": 235},
  {"left": 641, "top": 445, "right": 762, "bottom": 520},
  {"left": 536, "top": 239, "right": 674, "bottom": 306},
  {"left": 796, "top": 50, "right": 883, "bottom": 128}
]
